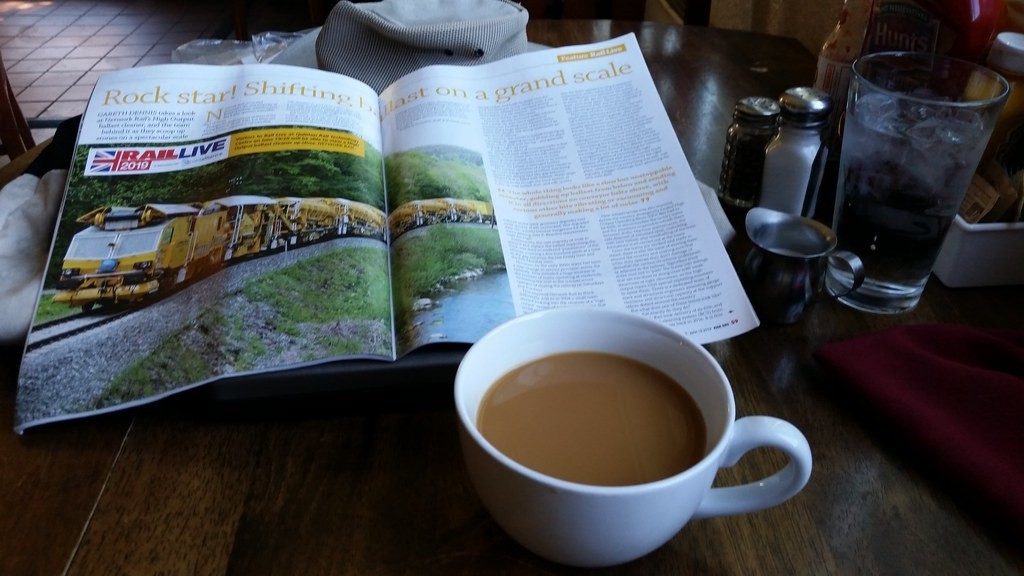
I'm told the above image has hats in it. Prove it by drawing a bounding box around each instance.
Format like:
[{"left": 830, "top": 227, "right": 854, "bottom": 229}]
[{"left": 268, "top": 0, "right": 552, "bottom": 97}]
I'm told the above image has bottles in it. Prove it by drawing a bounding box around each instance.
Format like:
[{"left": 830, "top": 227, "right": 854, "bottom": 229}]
[
  {"left": 718, "top": 95, "right": 781, "bottom": 210},
  {"left": 987, "top": 32, "right": 1024, "bottom": 165},
  {"left": 759, "top": 85, "right": 834, "bottom": 216}
]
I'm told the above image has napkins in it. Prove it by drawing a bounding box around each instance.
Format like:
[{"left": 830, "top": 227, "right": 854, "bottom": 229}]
[{"left": 809, "top": 323, "right": 1024, "bottom": 541}]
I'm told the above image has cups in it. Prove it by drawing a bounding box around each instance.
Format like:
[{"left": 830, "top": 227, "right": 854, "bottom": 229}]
[
  {"left": 823, "top": 51, "right": 1012, "bottom": 316},
  {"left": 452, "top": 304, "right": 813, "bottom": 568}
]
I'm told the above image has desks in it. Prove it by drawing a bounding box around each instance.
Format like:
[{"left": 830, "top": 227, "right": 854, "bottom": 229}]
[{"left": 0, "top": 19, "right": 1024, "bottom": 576}]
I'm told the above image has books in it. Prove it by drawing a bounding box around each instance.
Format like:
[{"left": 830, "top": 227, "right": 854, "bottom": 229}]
[{"left": 13, "top": 30, "right": 760, "bottom": 437}]
